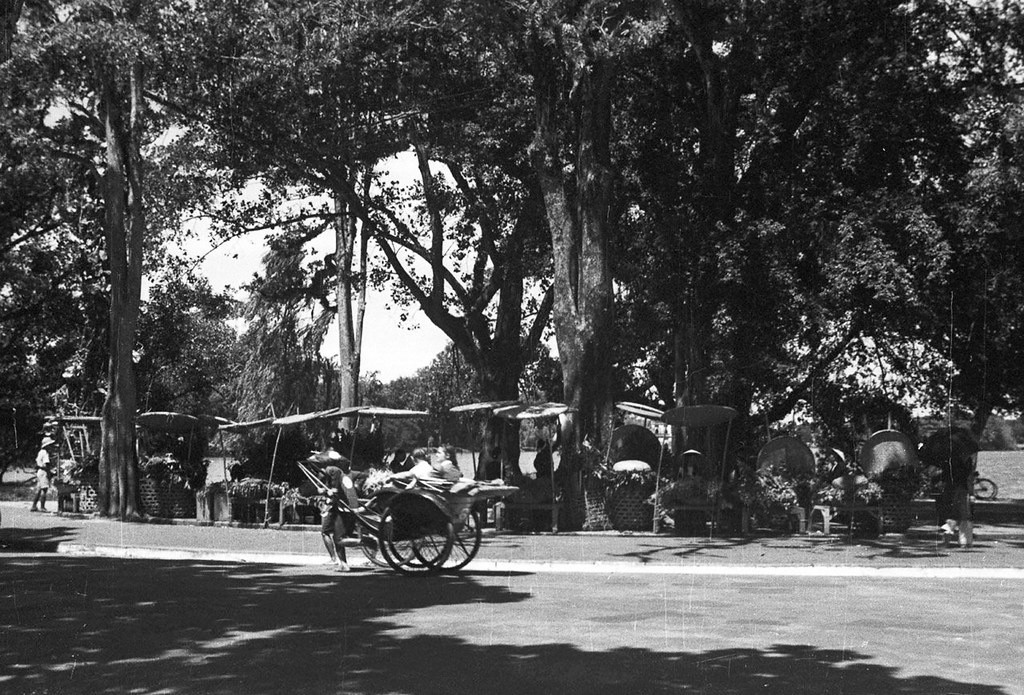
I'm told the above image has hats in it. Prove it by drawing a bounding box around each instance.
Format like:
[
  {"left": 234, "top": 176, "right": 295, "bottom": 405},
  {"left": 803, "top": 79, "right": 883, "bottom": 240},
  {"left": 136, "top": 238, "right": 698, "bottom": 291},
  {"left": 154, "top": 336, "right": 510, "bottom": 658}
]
[
  {"left": 41, "top": 436, "right": 56, "bottom": 449},
  {"left": 309, "top": 448, "right": 351, "bottom": 464}
]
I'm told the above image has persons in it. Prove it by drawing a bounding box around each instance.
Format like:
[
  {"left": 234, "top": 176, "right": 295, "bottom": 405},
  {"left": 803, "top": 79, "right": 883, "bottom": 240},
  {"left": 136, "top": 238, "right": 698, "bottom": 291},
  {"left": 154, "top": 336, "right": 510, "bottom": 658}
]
[
  {"left": 431, "top": 445, "right": 463, "bottom": 480},
  {"left": 675, "top": 449, "right": 703, "bottom": 480},
  {"left": 935, "top": 453, "right": 973, "bottom": 539},
  {"left": 307, "top": 447, "right": 356, "bottom": 573},
  {"left": 30, "top": 436, "right": 57, "bottom": 513},
  {"left": 388, "top": 450, "right": 406, "bottom": 473},
  {"left": 409, "top": 448, "right": 434, "bottom": 477},
  {"left": 731, "top": 457, "right": 751, "bottom": 486},
  {"left": 484, "top": 447, "right": 505, "bottom": 480},
  {"left": 230, "top": 461, "right": 270, "bottom": 483},
  {"left": 532, "top": 439, "right": 555, "bottom": 478}
]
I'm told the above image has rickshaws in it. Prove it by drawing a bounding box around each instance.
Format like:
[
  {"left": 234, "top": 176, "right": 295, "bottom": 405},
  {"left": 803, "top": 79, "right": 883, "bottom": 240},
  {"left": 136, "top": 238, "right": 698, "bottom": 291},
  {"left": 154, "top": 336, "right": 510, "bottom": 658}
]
[{"left": 299, "top": 459, "right": 520, "bottom": 577}]
